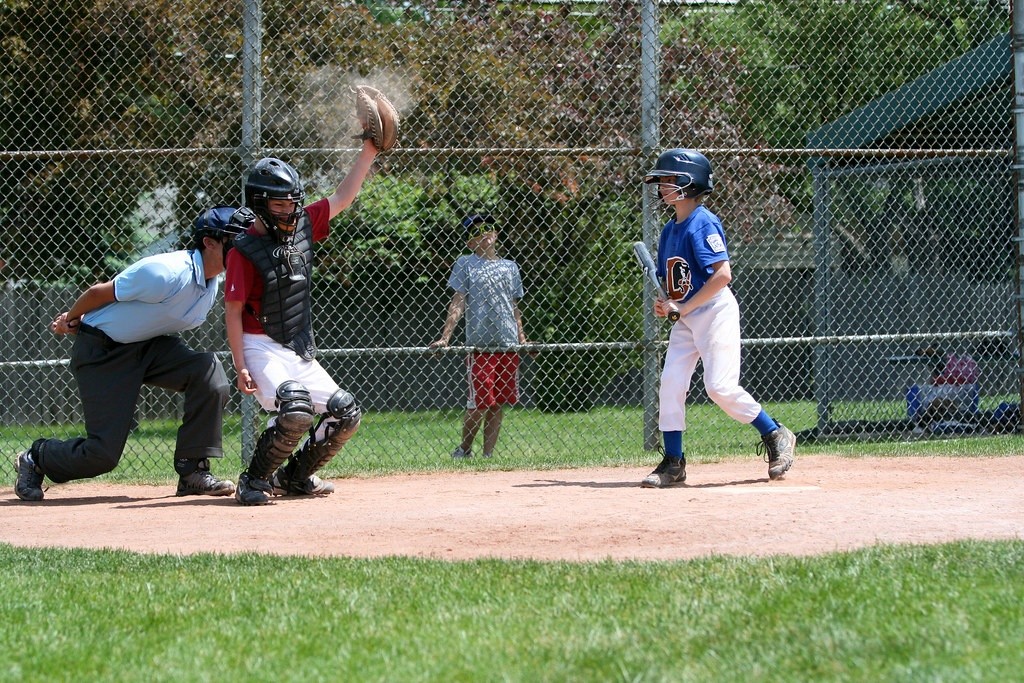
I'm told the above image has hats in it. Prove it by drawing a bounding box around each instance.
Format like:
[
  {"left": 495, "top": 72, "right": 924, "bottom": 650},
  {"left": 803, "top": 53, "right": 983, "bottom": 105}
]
[
  {"left": 464, "top": 216, "right": 495, "bottom": 236},
  {"left": 196, "top": 207, "right": 238, "bottom": 231}
]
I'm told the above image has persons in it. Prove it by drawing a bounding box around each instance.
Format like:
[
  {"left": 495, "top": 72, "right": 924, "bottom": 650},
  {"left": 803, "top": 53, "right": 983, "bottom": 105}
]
[
  {"left": 224, "top": 84, "right": 401, "bottom": 507},
  {"left": 432, "top": 215, "right": 540, "bottom": 456},
  {"left": 13, "top": 209, "right": 238, "bottom": 501},
  {"left": 641, "top": 148, "right": 796, "bottom": 487}
]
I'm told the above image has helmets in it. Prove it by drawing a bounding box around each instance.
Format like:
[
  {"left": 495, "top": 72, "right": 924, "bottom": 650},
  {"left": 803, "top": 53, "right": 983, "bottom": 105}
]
[
  {"left": 245, "top": 158, "right": 305, "bottom": 242},
  {"left": 645, "top": 148, "right": 713, "bottom": 207}
]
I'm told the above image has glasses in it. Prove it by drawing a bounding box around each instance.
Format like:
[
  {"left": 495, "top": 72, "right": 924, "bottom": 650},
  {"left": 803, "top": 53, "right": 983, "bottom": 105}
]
[{"left": 468, "top": 224, "right": 495, "bottom": 240}]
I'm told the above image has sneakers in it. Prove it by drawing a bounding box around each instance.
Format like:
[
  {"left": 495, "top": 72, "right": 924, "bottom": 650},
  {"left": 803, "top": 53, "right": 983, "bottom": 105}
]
[
  {"left": 641, "top": 446, "right": 686, "bottom": 487},
  {"left": 236, "top": 473, "right": 269, "bottom": 506},
  {"left": 176, "top": 459, "right": 236, "bottom": 495},
  {"left": 14, "top": 449, "right": 45, "bottom": 501},
  {"left": 756, "top": 418, "right": 796, "bottom": 479},
  {"left": 270, "top": 472, "right": 335, "bottom": 495}
]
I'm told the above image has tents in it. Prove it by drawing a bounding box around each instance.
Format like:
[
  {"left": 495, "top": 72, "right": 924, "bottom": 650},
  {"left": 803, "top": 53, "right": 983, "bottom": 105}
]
[{"left": 806, "top": 35, "right": 1024, "bottom": 436}]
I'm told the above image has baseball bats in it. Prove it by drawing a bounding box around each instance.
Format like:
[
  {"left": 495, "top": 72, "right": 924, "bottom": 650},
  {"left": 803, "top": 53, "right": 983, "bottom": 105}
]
[{"left": 633, "top": 241, "right": 680, "bottom": 322}]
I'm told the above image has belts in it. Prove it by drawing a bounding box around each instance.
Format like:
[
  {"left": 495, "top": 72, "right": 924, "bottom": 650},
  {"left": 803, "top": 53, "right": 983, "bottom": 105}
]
[{"left": 77, "top": 321, "right": 110, "bottom": 340}]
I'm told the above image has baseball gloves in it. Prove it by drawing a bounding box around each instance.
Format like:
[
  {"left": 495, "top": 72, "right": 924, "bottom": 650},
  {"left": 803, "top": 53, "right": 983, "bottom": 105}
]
[{"left": 346, "top": 85, "right": 402, "bottom": 153}]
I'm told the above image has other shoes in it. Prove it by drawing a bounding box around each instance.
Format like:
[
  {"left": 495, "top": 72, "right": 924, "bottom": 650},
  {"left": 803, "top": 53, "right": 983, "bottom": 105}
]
[{"left": 452, "top": 445, "right": 472, "bottom": 458}]
